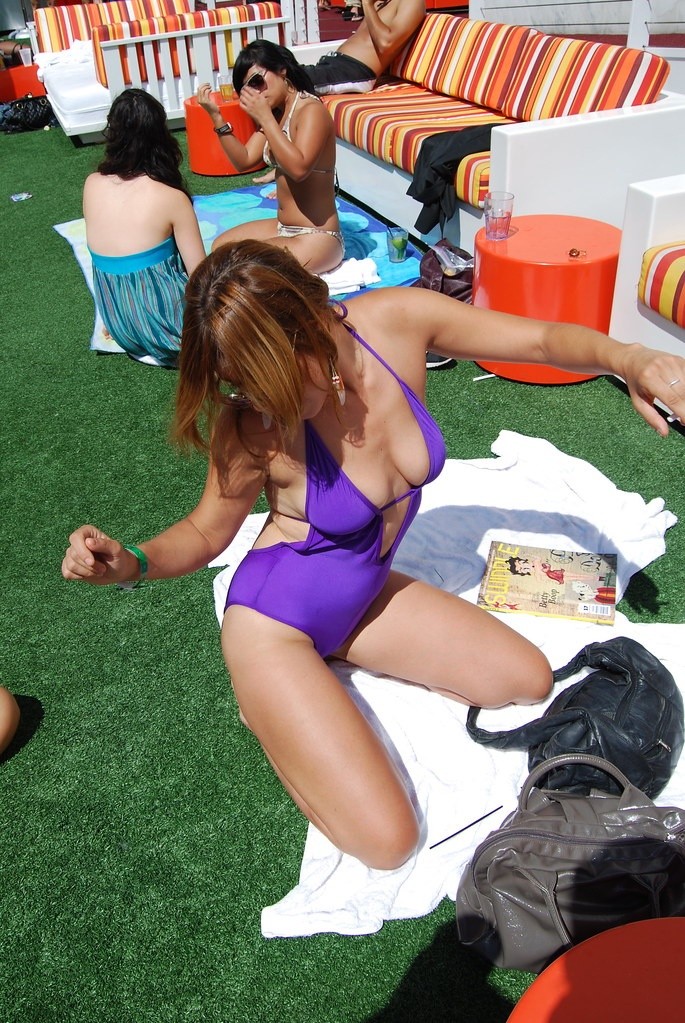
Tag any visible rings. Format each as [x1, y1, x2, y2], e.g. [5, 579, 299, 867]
[669, 379, 680, 387]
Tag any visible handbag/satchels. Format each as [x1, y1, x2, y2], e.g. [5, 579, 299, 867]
[0, 92, 55, 133]
[467, 636, 685, 795]
[409, 236, 475, 308]
[2, 43, 34, 68]
[453, 754, 685, 970]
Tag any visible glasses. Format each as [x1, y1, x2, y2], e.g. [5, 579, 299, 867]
[246, 68, 267, 89]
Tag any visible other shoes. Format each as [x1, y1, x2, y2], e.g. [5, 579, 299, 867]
[424, 352, 453, 368]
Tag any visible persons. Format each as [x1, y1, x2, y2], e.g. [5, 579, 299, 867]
[56, 239, 684, 869]
[82, 88, 210, 364]
[0, 40, 32, 58]
[251, 0, 427, 183]
[196, 37, 344, 272]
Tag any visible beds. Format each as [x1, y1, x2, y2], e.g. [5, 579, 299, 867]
[34, 0, 289, 148]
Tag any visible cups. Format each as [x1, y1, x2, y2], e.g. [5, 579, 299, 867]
[484, 191, 514, 241]
[386, 227, 409, 264]
[218, 75, 234, 103]
[19, 48, 32, 67]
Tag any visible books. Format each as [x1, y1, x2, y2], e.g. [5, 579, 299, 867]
[476, 540, 619, 625]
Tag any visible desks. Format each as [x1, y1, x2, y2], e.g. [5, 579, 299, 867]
[185, 90, 268, 175]
[473, 213, 624, 386]
[0, 62, 46, 103]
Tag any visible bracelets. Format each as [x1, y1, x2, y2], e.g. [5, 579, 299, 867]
[120, 546, 148, 589]
[214, 122, 234, 133]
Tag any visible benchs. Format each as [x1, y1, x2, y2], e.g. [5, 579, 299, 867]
[606, 174, 685, 431]
[286, 10, 684, 265]
